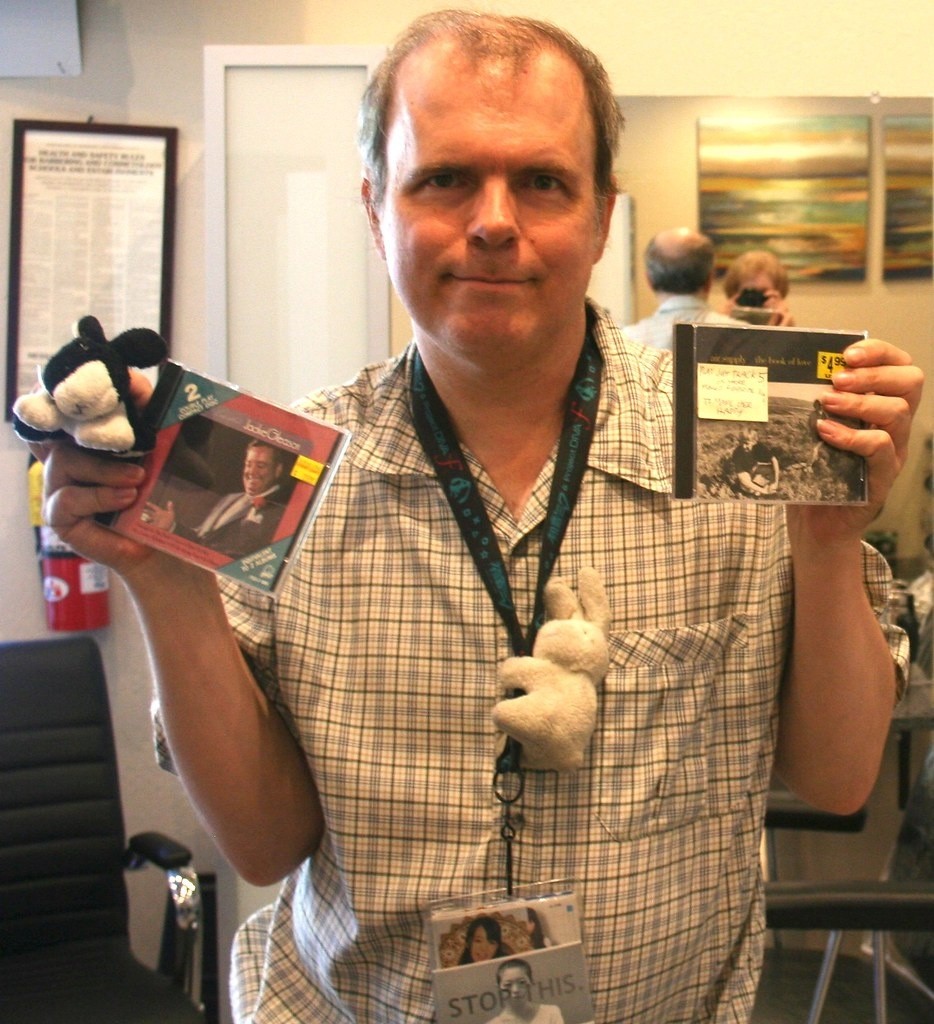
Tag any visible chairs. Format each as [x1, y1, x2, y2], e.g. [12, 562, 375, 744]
[890, 606, 934, 810]
[1, 635, 221, 1024]
[748, 741, 934, 1024]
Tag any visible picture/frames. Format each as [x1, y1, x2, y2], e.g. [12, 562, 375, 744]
[6, 119, 178, 426]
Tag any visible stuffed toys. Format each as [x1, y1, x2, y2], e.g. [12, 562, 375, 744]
[12, 315, 167, 464]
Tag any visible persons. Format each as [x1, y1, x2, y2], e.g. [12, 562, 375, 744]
[143, 438, 296, 559]
[892, 532, 934, 991]
[722, 400, 830, 494]
[618, 228, 799, 351]
[26, 8, 925, 1024]
[457, 908, 566, 1024]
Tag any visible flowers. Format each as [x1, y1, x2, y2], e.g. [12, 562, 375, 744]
[247, 496, 266, 524]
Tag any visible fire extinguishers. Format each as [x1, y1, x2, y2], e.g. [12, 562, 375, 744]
[27, 450, 114, 632]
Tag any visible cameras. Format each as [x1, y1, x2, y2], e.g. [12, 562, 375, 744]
[736, 289, 769, 307]
